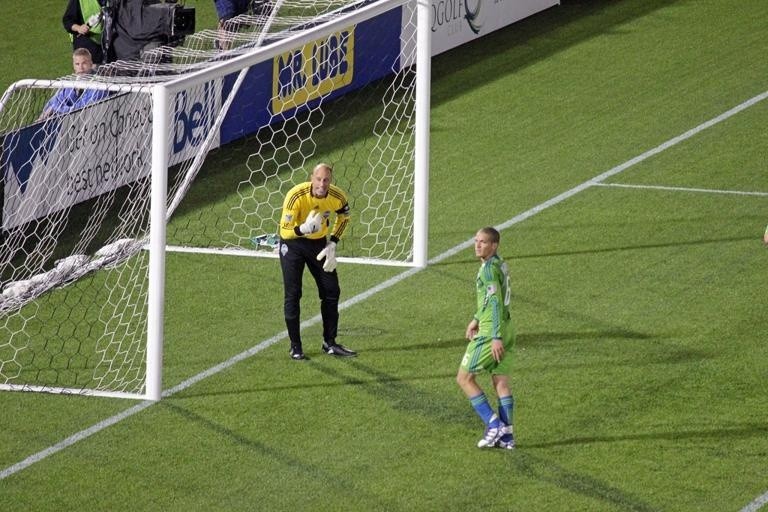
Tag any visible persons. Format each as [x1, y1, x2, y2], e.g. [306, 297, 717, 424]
[210, 1, 257, 55]
[456, 224, 517, 452]
[61, 1, 117, 70]
[277, 163, 359, 361]
[34, 48, 110, 121]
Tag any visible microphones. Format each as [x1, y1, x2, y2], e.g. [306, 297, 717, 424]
[76, 12, 103, 39]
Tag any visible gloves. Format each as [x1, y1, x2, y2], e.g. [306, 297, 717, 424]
[316, 240, 338, 273]
[299, 210, 323, 235]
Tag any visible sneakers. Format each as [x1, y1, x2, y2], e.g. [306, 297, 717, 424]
[477, 418, 516, 450]
[288, 347, 305, 360]
[321, 338, 358, 358]
